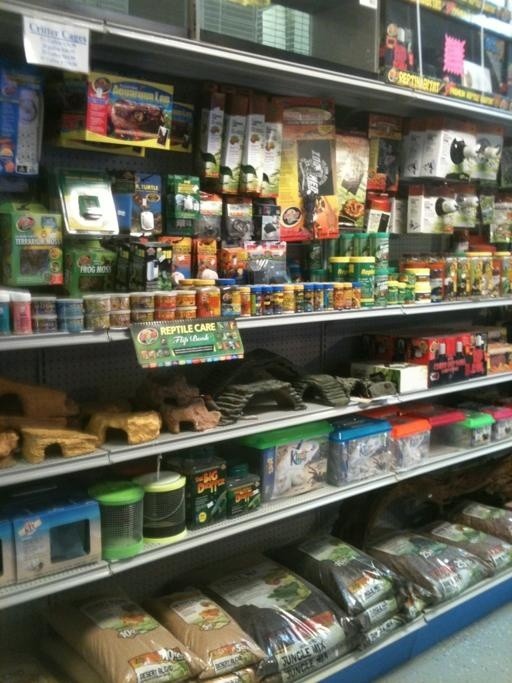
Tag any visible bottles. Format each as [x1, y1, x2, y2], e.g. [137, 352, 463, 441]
[87, 480, 145, 560]
[135, 470, 186, 544]
[0, 231, 512, 336]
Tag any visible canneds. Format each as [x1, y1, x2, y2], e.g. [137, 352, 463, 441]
[310, 232, 416, 308]
[154, 291, 177, 309]
[155, 308, 176, 320]
[131, 292, 155, 309]
[176, 280, 202, 290]
[32, 314, 58, 334]
[59, 316, 85, 333]
[131, 310, 154, 322]
[109, 310, 131, 328]
[0, 285, 33, 337]
[83, 294, 111, 314]
[231, 281, 361, 317]
[403, 251, 512, 303]
[56, 299, 84, 317]
[31, 293, 57, 315]
[176, 306, 198, 320]
[214, 279, 240, 320]
[84, 314, 111, 330]
[109, 293, 132, 310]
[175, 290, 197, 306]
[188, 280, 221, 318]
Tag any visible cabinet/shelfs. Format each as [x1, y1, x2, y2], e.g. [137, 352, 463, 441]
[0, 1, 110, 683]
[110, 0, 512, 683]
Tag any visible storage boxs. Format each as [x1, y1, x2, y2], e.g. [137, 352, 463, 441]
[352, 361, 428, 396]
[0, 519, 17, 587]
[483, 406, 512, 442]
[460, 411, 496, 447]
[404, 403, 466, 457]
[356, 406, 431, 473]
[60, 71, 175, 151]
[10, 485, 101, 585]
[364, 327, 488, 386]
[232, 420, 335, 502]
[326, 417, 393, 488]
